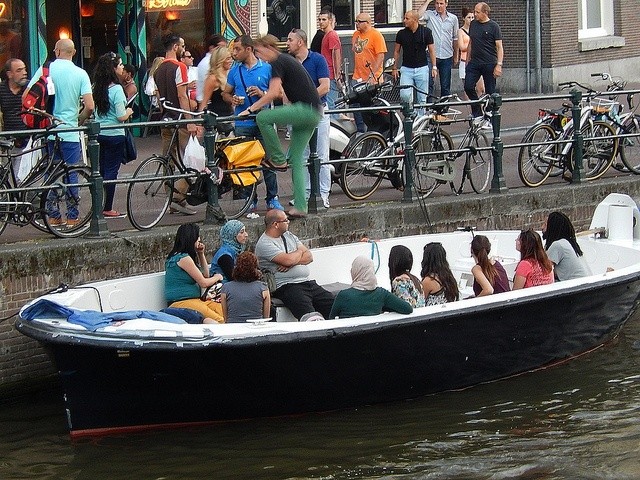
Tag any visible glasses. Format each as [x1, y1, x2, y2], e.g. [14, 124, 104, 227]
[276, 218, 288, 222]
[110, 52, 116, 61]
[291, 28, 305, 43]
[318, 18, 328, 21]
[355, 19, 369, 22]
[182, 56, 191, 58]
[177, 44, 184, 47]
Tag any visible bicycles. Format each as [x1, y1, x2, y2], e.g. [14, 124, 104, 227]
[518, 72, 639, 187]
[0, 108, 106, 238]
[399, 85, 492, 200]
[341, 85, 458, 201]
[127, 97, 255, 230]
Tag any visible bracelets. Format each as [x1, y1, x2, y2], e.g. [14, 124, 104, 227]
[432, 65, 438, 70]
[497, 62, 503, 67]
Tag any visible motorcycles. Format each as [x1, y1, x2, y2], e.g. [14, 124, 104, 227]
[285, 57, 403, 188]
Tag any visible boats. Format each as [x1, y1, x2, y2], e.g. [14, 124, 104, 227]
[15, 193, 640, 438]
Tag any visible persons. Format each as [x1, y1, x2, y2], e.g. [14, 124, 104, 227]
[286, 29, 331, 209]
[421, 242, 461, 306]
[91, 52, 135, 220]
[350, 12, 388, 137]
[464, 2, 505, 129]
[221, 251, 271, 323]
[177, 49, 198, 112]
[0, 58, 42, 223]
[237, 35, 323, 220]
[209, 219, 248, 284]
[255, 209, 336, 322]
[310, 12, 337, 54]
[458, 7, 487, 118]
[119, 64, 139, 103]
[415, 0, 459, 113]
[463, 234, 511, 299]
[388, 245, 426, 309]
[318, 10, 344, 123]
[153, 32, 199, 215]
[198, 46, 246, 201]
[21, 38, 95, 228]
[164, 222, 224, 324]
[391, 10, 438, 128]
[329, 256, 413, 318]
[145, 57, 164, 109]
[196, 34, 227, 111]
[512, 228, 555, 291]
[222, 34, 285, 216]
[541, 211, 591, 282]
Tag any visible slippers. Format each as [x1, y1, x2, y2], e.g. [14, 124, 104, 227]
[260, 159, 288, 172]
[286, 211, 308, 218]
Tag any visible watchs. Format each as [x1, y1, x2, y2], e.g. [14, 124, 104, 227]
[246, 106, 252, 114]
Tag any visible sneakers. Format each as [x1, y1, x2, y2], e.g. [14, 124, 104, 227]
[48, 217, 66, 227]
[240, 196, 256, 213]
[67, 219, 84, 229]
[473, 116, 483, 126]
[170, 198, 197, 216]
[267, 196, 285, 211]
[102, 209, 120, 215]
[480, 118, 494, 130]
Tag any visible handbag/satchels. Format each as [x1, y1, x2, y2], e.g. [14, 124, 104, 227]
[200, 281, 224, 302]
[21, 60, 55, 129]
[260, 268, 277, 294]
[122, 120, 137, 165]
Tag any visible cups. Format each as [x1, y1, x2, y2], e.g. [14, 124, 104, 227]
[235, 96, 245, 104]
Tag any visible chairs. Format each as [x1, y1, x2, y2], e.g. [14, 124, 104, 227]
[123, 91, 140, 137]
[141, 81, 167, 138]
